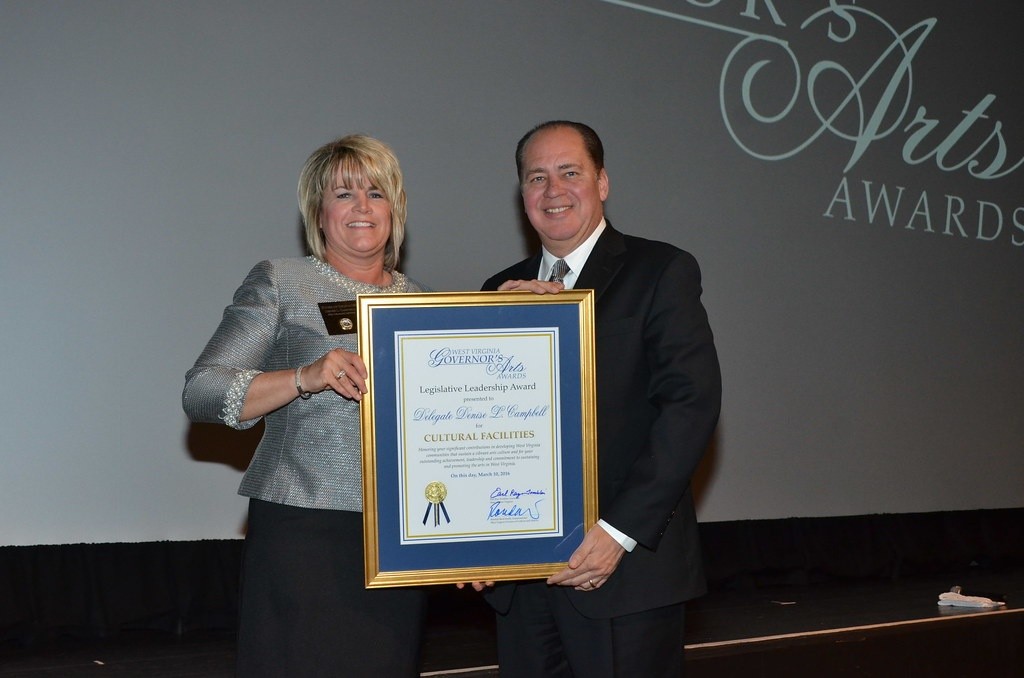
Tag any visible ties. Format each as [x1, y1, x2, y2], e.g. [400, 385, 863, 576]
[547, 259, 571, 286]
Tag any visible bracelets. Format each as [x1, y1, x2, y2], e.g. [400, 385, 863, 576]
[294, 364, 312, 400]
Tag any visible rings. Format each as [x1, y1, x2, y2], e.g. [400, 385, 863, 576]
[335, 370, 347, 381]
[589, 579, 597, 588]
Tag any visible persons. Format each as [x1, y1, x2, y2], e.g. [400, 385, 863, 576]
[479, 118, 723, 677]
[182, 134, 495, 678]
